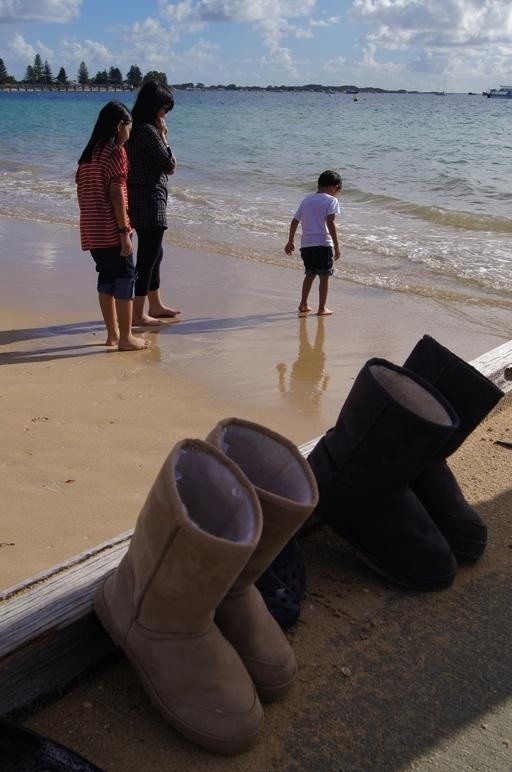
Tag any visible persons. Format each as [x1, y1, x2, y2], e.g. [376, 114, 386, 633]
[284, 170, 342, 316]
[74, 99, 151, 351]
[123, 80, 181, 325]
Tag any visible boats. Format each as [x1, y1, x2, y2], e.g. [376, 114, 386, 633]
[434, 91, 448, 96]
[486, 84, 512, 99]
[467, 92, 477, 95]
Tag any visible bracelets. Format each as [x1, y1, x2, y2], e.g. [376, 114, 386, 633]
[119, 226, 130, 234]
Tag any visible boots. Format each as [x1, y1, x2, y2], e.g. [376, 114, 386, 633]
[301, 354, 463, 593]
[398, 329, 507, 569]
[202, 400, 322, 695]
[96, 434, 265, 752]
